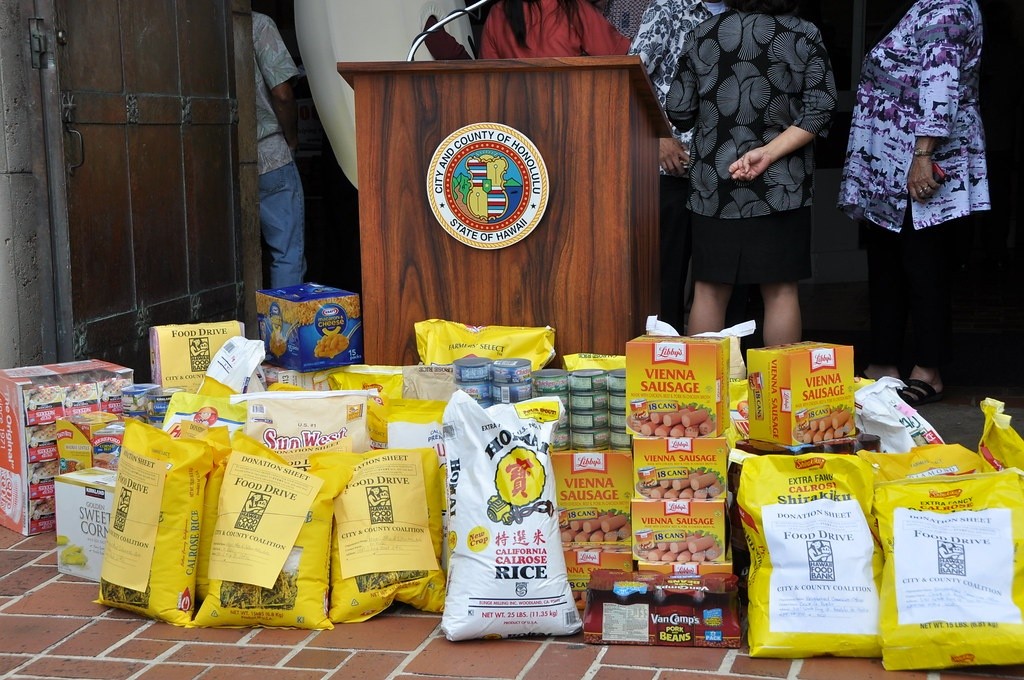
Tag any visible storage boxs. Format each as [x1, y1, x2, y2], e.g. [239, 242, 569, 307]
[548, 336, 858, 607]
[253, 280, 364, 392]
[0, 359, 135, 582]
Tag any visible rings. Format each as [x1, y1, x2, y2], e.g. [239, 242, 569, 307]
[922, 187, 929, 191]
[916, 190, 923, 195]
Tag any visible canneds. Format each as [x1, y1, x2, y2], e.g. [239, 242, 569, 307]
[453, 358, 632, 452]
[120, 385, 185, 430]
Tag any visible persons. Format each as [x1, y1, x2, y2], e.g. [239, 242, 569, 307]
[478, 0, 631, 60]
[666, 0, 837, 346]
[626, 0, 714, 335]
[252, 13, 307, 291]
[836, 0, 994, 405]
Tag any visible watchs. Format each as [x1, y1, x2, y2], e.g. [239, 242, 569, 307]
[913, 149, 933, 156]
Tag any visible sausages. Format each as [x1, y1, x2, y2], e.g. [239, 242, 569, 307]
[560, 405, 858, 563]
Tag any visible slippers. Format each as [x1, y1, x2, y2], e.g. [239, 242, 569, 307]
[897, 379, 942, 406]
[854, 370, 869, 379]
